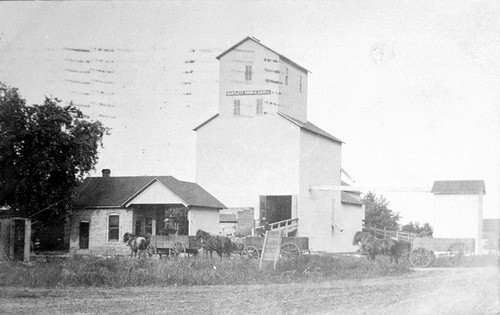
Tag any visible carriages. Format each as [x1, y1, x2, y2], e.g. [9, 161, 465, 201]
[123, 232, 203, 260]
[198, 229, 309, 263]
[353, 230, 474, 269]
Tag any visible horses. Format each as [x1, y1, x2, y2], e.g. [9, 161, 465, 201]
[195, 229, 232, 263]
[123, 232, 148, 259]
[352, 230, 401, 265]
